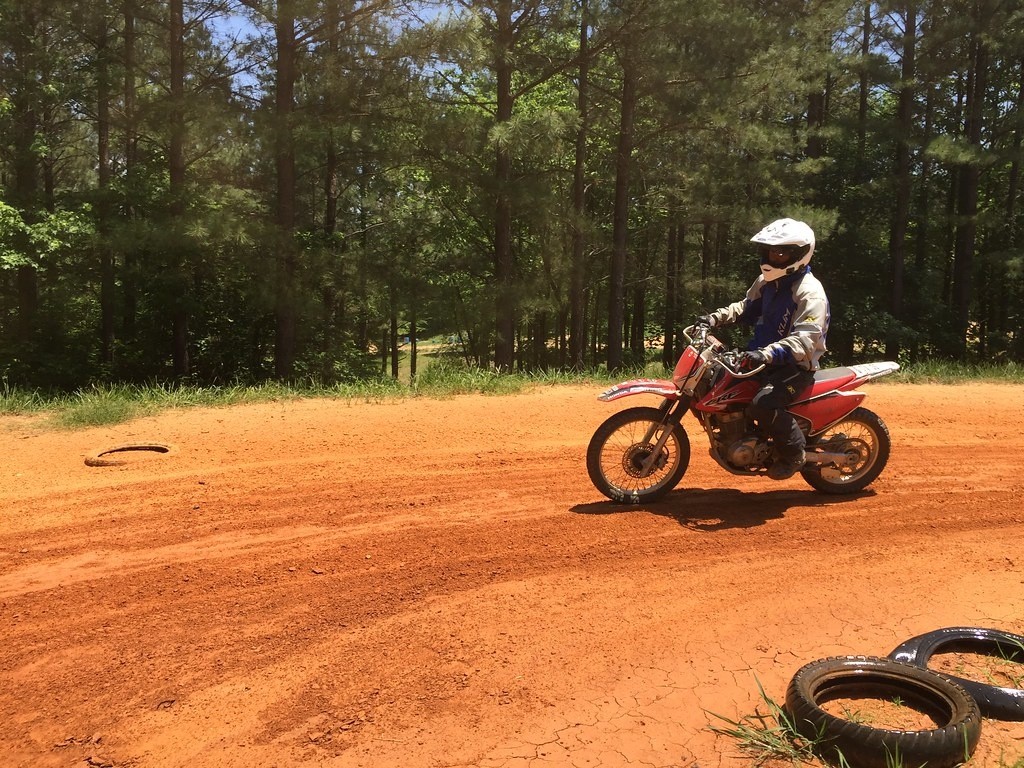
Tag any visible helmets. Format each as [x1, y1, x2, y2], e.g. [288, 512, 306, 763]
[749, 218, 815, 283]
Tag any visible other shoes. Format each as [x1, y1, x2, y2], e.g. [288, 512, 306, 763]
[765, 450, 806, 480]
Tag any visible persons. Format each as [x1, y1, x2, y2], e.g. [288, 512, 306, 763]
[695, 219, 829, 480]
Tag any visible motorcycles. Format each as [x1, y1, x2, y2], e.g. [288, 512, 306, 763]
[585, 316, 902, 507]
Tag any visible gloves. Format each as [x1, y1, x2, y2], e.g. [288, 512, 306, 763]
[691, 314, 712, 337]
[731, 349, 768, 372]
